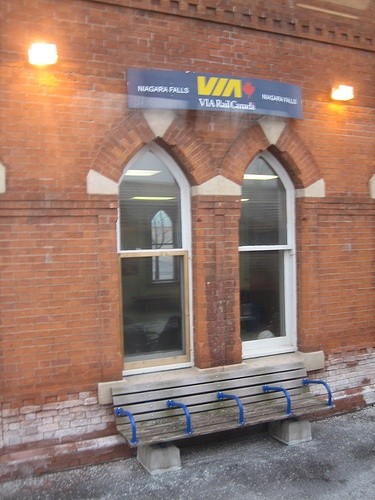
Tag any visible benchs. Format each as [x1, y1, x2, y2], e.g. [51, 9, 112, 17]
[110, 362, 336, 475]
